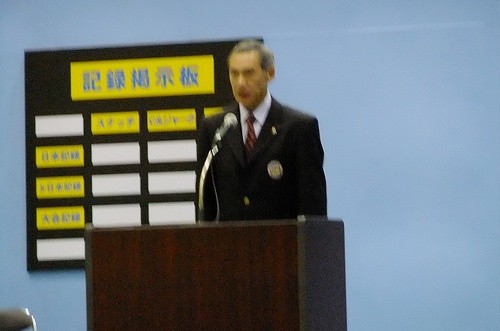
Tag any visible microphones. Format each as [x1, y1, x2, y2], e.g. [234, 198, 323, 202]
[213, 112, 238, 147]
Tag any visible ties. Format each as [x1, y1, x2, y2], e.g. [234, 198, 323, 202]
[244, 112, 256, 163]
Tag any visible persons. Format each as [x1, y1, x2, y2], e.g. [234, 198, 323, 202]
[194, 39, 327, 224]
